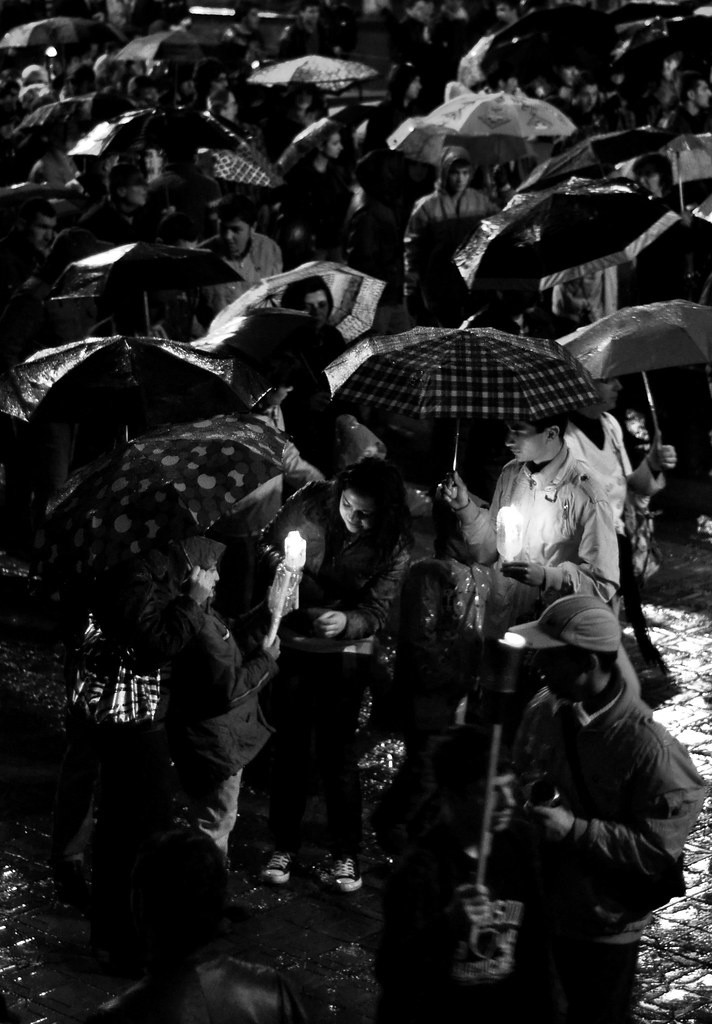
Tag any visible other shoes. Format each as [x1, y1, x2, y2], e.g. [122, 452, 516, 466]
[223, 905, 252, 925]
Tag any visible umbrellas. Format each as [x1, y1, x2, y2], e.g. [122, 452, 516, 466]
[617, 2, 709, 57]
[247, 55, 381, 92]
[420, 93, 579, 140]
[455, 3, 614, 93]
[5, 16, 123, 82]
[553, 298, 709, 451]
[320, 324, 607, 500]
[206, 259, 390, 385]
[35, 422, 291, 579]
[512, 129, 682, 192]
[202, 109, 287, 187]
[63, 104, 242, 159]
[40, 241, 249, 335]
[112, 27, 250, 67]
[4, 335, 276, 425]
[451, 174, 686, 293]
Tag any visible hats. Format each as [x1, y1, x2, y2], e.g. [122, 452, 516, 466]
[504, 592, 623, 654]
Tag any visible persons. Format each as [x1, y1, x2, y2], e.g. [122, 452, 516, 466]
[258, 453, 412, 894]
[566, 377, 683, 711]
[496, 593, 689, 1024]
[2, 1, 711, 399]
[434, 403, 622, 628]
[36, 538, 275, 981]
[83, 828, 308, 1024]
[374, 725, 571, 1022]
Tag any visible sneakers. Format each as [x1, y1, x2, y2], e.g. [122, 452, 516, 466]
[263, 851, 292, 884]
[334, 856, 363, 891]
